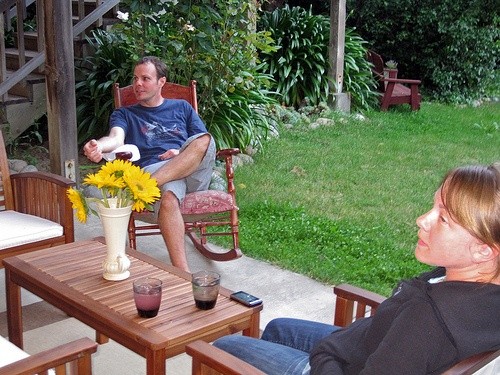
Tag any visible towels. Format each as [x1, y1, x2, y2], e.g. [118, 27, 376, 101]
[101, 143, 141, 163]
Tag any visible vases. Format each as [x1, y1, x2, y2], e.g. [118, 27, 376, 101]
[98, 198, 133, 281]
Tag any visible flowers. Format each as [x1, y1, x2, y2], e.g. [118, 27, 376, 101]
[66, 157, 161, 223]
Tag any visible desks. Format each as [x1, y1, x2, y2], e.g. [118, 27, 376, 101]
[0, 234, 265, 375]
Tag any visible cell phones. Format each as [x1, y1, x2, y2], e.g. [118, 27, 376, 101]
[230, 291, 262, 307]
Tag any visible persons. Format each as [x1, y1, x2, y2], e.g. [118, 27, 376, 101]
[83, 56, 216, 275]
[213, 163, 500, 374]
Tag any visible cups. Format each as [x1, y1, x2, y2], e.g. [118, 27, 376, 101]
[191, 270, 221, 310]
[132, 278, 162, 318]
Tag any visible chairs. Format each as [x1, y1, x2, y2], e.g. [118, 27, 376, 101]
[369, 52, 423, 113]
[185, 282, 499, 375]
[0, 130, 76, 318]
[115, 81, 243, 262]
[1, 334, 98, 375]
